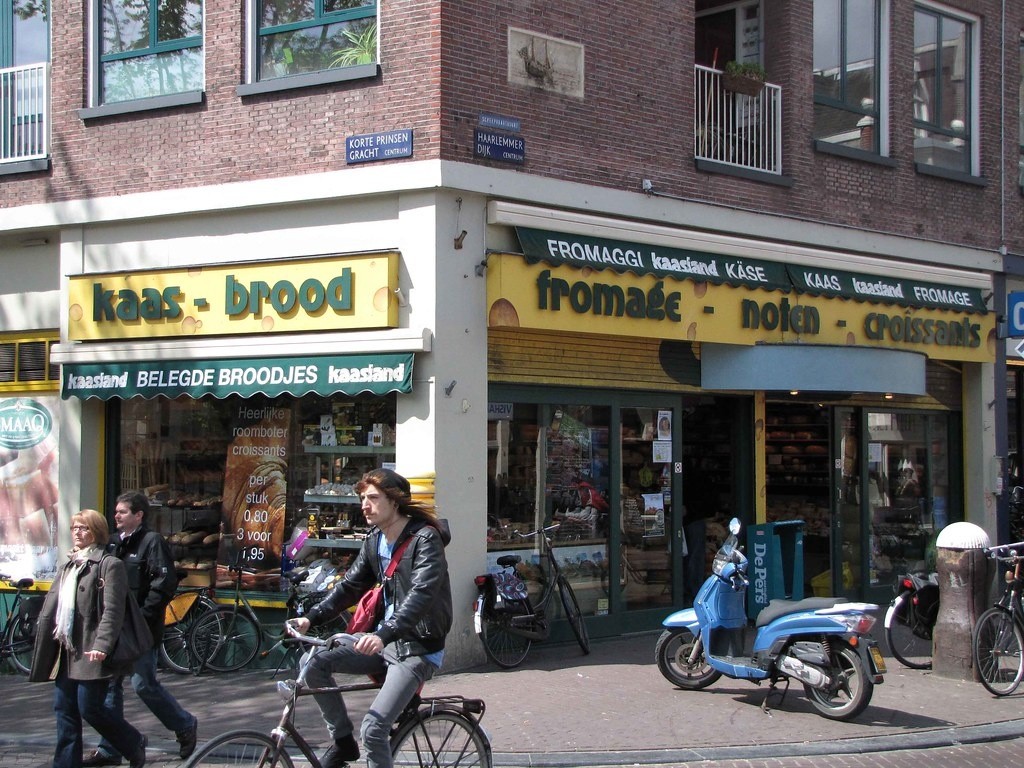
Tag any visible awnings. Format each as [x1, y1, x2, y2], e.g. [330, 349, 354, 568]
[47, 327, 432, 398]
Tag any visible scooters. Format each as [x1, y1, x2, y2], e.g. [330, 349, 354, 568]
[654, 518, 889, 722]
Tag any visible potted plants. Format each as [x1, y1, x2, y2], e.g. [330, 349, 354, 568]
[721, 60, 768, 97]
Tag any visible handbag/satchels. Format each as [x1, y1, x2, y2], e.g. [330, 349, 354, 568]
[344, 584, 384, 635]
[891, 574, 910, 627]
[19, 596, 45, 638]
[908, 574, 940, 640]
[481, 572, 528, 620]
[95, 554, 154, 668]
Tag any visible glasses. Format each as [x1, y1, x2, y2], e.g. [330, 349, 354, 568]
[70, 525, 89, 532]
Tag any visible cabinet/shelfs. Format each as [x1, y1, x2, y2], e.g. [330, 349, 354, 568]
[545, 426, 612, 541]
[140, 448, 229, 588]
[764, 423, 831, 487]
[302, 445, 395, 550]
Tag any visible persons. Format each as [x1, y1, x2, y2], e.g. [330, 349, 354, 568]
[26, 489, 198, 768]
[893, 464, 928, 498]
[681, 457, 707, 604]
[289, 471, 453, 767]
[570, 476, 609, 537]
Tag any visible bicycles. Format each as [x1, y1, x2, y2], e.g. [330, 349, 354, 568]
[971, 541, 1024, 698]
[186, 543, 353, 683]
[884, 574, 939, 670]
[470, 520, 590, 668]
[157, 568, 224, 677]
[183, 617, 493, 768]
[991, 485, 1024, 542]
[601, 539, 671, 598]
[0, 573, 47, 676]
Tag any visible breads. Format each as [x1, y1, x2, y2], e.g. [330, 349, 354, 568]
[142, 483, 223, 571]
[222, 406, 292, 560]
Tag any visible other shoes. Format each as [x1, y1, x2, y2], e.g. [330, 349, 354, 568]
[83, 750, 122, 767]
[312, 740, 360, 768]
[129, 734, 148, 768]
[176, 719, 197, 759]
